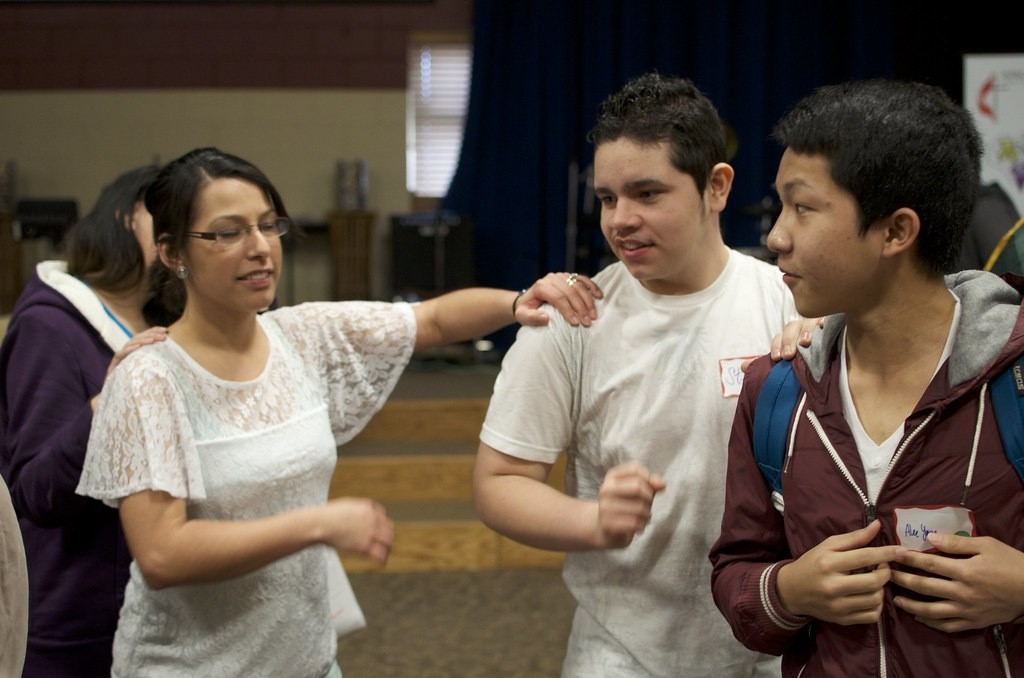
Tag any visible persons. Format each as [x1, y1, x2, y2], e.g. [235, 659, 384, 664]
[108, 142, 604, 677]
[708, 83, 1023, 677]
[0, 165, 169, 678]
[470, 69, 827, 678]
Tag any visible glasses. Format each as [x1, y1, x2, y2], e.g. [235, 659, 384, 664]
[177, 216, 291, 253]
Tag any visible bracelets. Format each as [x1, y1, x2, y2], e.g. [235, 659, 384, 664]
[512, 287, 531, 319]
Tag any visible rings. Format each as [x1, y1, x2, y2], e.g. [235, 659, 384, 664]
[566, 271, 579, 287]
